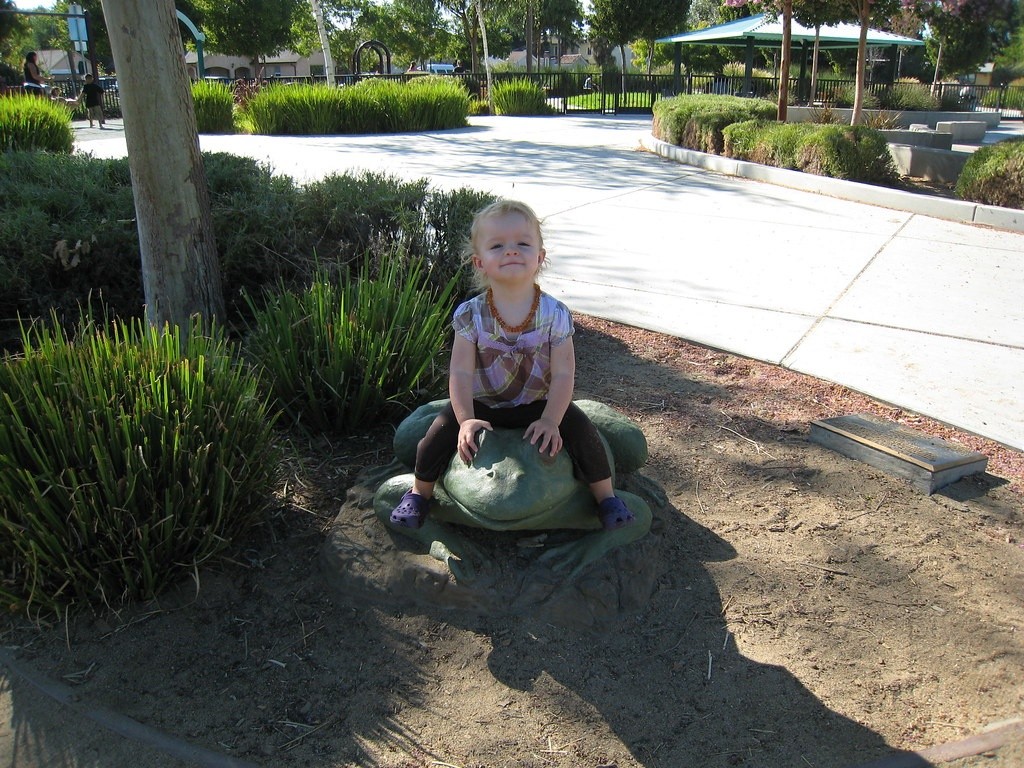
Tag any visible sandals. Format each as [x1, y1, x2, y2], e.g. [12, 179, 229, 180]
[597, 494, 635, 532]
[390, 486, 434, 529]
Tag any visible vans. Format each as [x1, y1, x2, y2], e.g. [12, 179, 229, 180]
[417, 64, 455, 74]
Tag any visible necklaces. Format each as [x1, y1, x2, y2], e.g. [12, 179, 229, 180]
[486, 284, 540, 332]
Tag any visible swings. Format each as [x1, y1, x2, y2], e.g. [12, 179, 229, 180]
[31, 20, 79, 109]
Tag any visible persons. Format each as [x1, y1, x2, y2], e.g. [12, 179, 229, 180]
[77, 74, 105, 128]
[407, 61, 419, 72]
[50, 87, 62, 101]
[24, 52, 51, 96]
[453, 60, 466, 73]
[584, 74, 596, 94]
[389, 200, 635, 531]
[954, 78, 976, 103]
